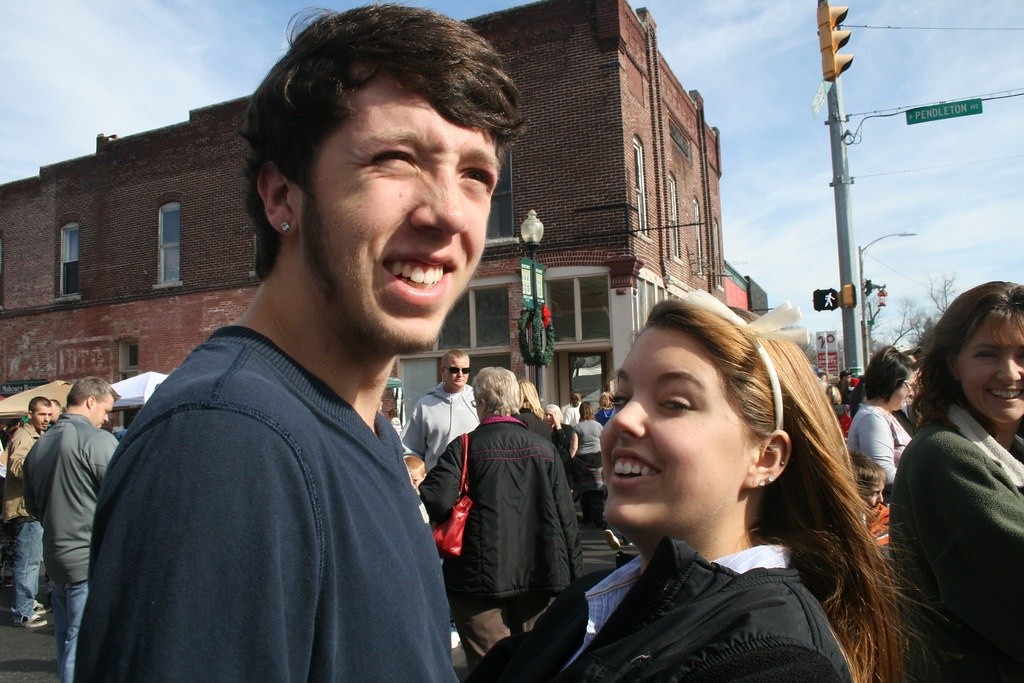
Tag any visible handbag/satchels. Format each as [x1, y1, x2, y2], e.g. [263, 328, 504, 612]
[431, 433, 473, 556]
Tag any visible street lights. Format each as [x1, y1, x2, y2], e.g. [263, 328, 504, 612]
[861, 230, 918, 370]
[520, 208, 546, 412]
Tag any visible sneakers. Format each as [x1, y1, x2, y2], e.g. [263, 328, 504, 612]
[14, 614, 48, 627]
[34, 603, 53, 614]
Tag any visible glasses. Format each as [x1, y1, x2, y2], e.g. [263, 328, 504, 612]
[903, 381, 918, 389]
[441, 367, 470, 374]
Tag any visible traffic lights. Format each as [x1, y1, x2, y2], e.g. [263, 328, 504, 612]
[813, 289, 838, 314]
[817, 0, 855, 83]
[837, 284, 858, 309]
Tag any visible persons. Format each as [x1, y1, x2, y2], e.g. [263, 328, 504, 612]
[71, 4, 533, 683]
[0, 280, 1024, 683]
[461, 296, 959, 683]
